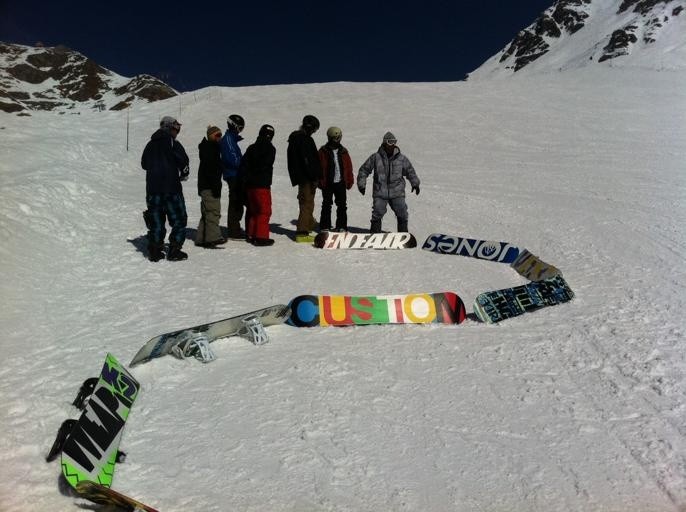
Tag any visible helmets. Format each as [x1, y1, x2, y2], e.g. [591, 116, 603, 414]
[227, 114, 245, 128]
[302, 115, 320, 128]
[326, 127, 342, 137]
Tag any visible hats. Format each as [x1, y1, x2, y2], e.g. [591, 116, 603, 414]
[259, 124, 275, 135]
[207, 126, 220, 136]
[383, 132, 397, 143]
[159, 116, 182, 130]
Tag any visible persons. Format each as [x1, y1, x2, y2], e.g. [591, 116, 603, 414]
[241, 123, 280, 248]
[139, 117, 189, 261]
[357, 131, 421, 233]
[287, 115, 322, 243]
[222, 114, 247, 242]
[198, 124, 227, 249]
[314, 127, 356, 235]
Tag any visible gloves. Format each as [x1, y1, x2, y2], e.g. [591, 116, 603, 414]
[411, 186, 420, 195]
[358, 187, 365, 195]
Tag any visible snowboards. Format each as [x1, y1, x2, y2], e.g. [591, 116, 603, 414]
[284, 291, 466, 325]
[64, 352, 140, 494]
[509, 247, 563, 283]
[75, 478, 158, 512]
[128, 303, 292, 369]
[421, 233, 519, 264]
[472, 275, 574, 324]
[314, 231, 417, 250]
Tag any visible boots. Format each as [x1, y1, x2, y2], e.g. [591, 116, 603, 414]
[148, 244, 188, 262]
[371, 224, 410, 232]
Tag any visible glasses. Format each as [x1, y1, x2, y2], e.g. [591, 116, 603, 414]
[384, 139, 397, 145]
[215, 133, 223, 137]
[329, 136, 341, 141]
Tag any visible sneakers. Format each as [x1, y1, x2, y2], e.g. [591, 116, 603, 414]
[320, 227, 351, 232]
[227, 229, 247, 238]
[248, 236, 275, 246]
[295, 232, 315, 243]
[195, 236, 228, 249]
